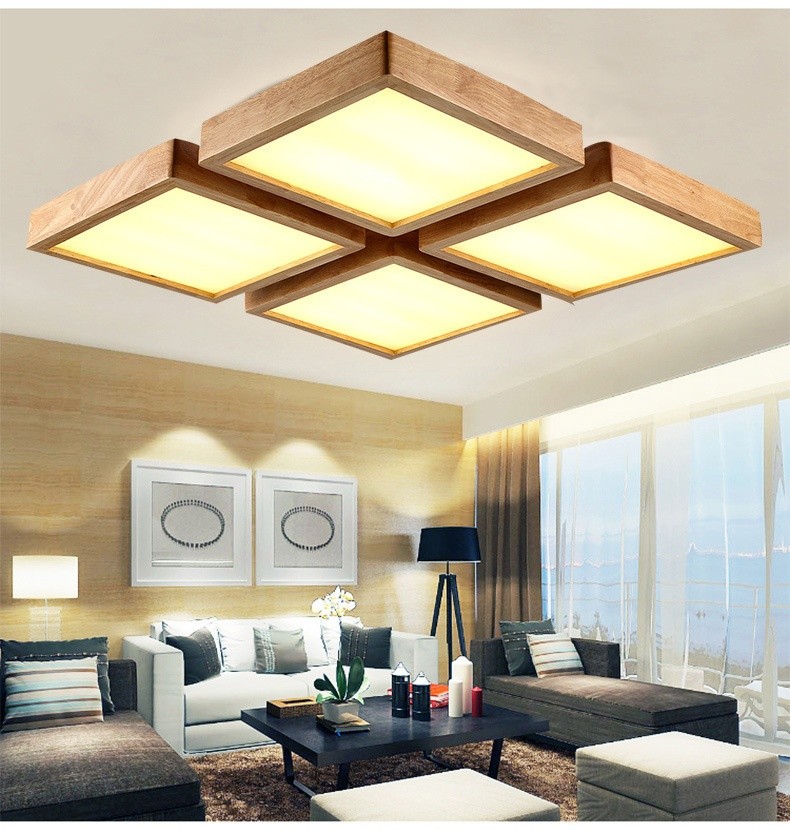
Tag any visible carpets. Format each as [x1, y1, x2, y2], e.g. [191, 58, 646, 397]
[182, 736, 790, 821]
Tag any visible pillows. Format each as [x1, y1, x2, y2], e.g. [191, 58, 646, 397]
[166, 628, 222, 690]
[252, 627, 310, 674]
[527, 634, 585, 679]
[498, 618, 555, 675]
[339, 623, 394, 671]
[0, 635, 115, 718]
[6, 656, 106, 731]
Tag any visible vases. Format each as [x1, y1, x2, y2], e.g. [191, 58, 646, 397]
[321, 701, 360, 723]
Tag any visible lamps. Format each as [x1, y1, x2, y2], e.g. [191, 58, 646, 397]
[13, 556, 79, 642]
[417, 526, 482, 680]
[26, 32, 761, 361]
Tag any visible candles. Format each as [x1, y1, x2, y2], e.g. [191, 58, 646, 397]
[392, 657, 483, 722]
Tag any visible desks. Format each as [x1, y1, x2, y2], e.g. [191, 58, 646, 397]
[241, 694, 550, 794]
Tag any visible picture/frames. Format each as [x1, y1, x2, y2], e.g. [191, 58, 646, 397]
[255, 470, 357, 585]
[131, 459, 252, 587]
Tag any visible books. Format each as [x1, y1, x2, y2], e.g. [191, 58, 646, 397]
[316, 715, 372, 734]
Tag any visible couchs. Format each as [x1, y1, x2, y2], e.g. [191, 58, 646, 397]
[121, 616, 440, 761]
[0, 660, 206, 821]
[470, 638, 739, 747]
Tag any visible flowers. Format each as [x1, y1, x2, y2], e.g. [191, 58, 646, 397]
[310, 584, 375, 706]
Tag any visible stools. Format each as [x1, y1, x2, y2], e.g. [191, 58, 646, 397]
[310, 766, 561, 822]
[576, 732, 778, 821]
